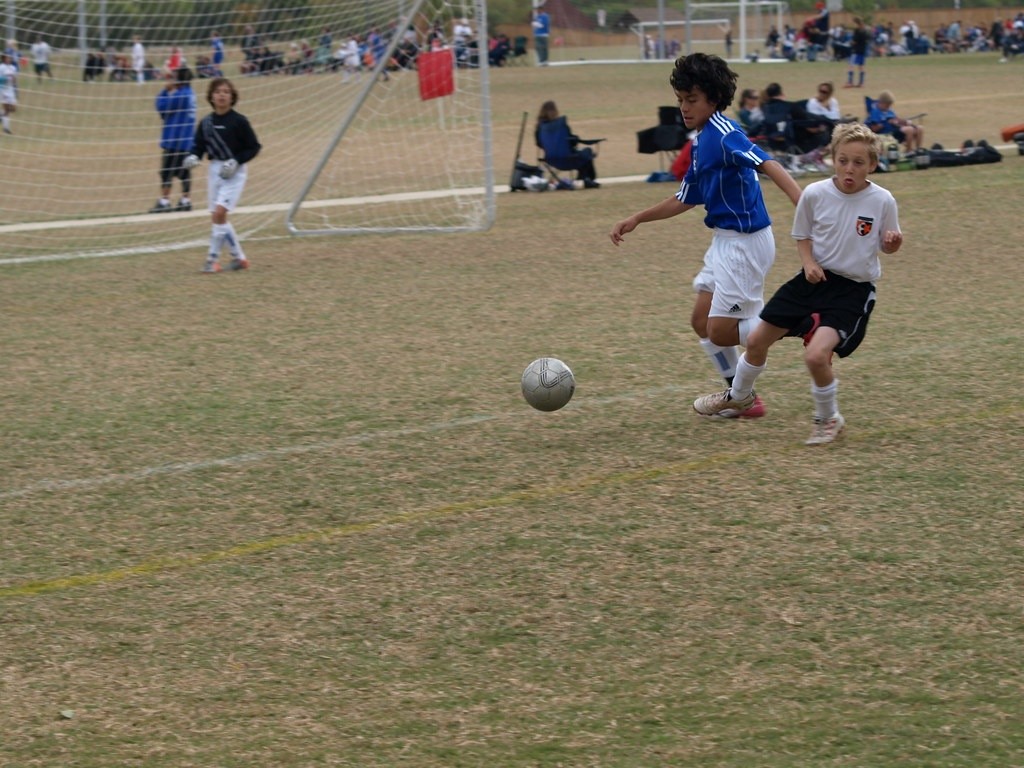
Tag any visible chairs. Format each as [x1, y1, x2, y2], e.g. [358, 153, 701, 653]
[866, 97, 926, 153]
[636, 105, 690, 173]
[741, 99, 860, 181]
[534, 124, 606, 192]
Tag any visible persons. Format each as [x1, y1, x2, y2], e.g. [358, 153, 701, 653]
[30, 34, 53, 85]
[182, 77, 263, 274]
[531, 7, 550, 67]
[83, 30, 226, 82]
[532, 101, 601, 187]
[725, 29, 733, 57]
[149, 67, 196, 213]
[692, 125, 904, 446]
[645, 34, 680, 59]
[239, 18, 510, 76]
[739, 82, 926, 152]
[0, 38, 22, 136]
[766, 0, 1024, 63]
[844, 15, 867, 89]
[747, 50, 760, 63]
[609, 52, 804, 425]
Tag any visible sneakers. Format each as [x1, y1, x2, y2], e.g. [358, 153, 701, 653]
[803, 312, 819, 348]
[806, 413, 845, 445]
[710, 396, 766, 418]
[693, 386, 755, 415]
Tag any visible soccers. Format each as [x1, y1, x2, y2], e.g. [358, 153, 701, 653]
[521, 358, 576, 412]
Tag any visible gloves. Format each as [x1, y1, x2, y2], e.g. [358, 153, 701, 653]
[219, 159, 239, 179]
[183, 154, 199, 170]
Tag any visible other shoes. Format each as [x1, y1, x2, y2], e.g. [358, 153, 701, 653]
[200, 262, 225, 273]
[150, 197, 172, 213]
[230, 257, 251, 270]
[2, 127, 12, 134]
[175, 198, 192, 212]
[584, 179, 601, 189]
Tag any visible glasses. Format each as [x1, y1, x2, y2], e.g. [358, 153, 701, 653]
[749, 96, 758, 100]
[819, 89, 828, 94]
